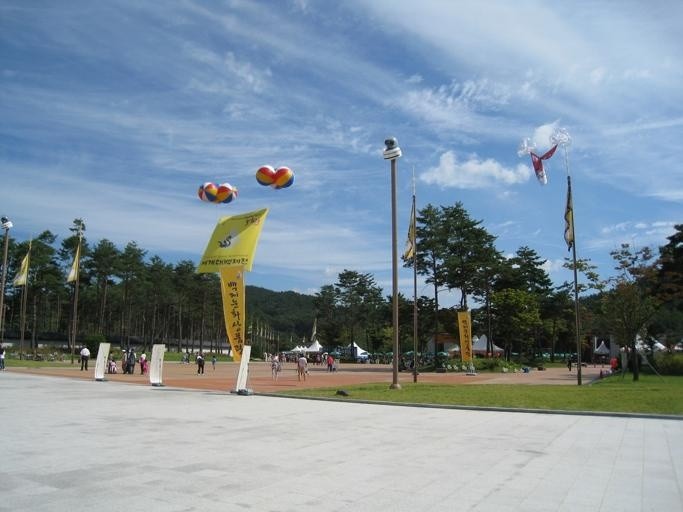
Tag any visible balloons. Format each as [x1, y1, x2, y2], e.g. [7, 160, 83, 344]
[197, 181, 238, 206]
[255, 165, 296, 190]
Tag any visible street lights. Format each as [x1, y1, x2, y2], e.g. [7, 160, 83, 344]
[383, 136, 401, 390]
[1, 217, 13, 325]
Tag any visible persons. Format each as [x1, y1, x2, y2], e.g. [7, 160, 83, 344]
[0, 348, 7, 371]
[183, 352, 205, 376]
[80, 345, 90, 371]
[566, 356, 572, 372]
[107, 348, 147, 376]
[262, 352, 337, 382]
[211, 356, 216, 370]
[610, 355, 618, 373]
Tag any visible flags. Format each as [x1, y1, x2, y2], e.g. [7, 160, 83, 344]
[564, 183, 575, 252]
[66, 243, 81, 284]
[402, 200, 416, 262]
[12, 251, 30, 288]
